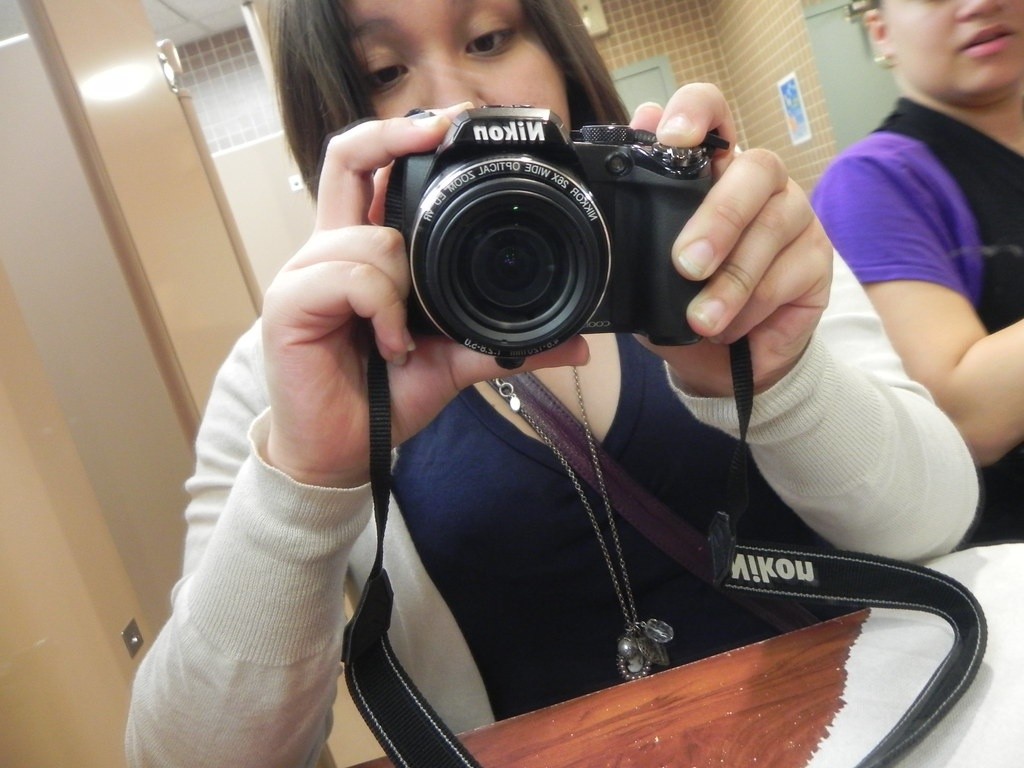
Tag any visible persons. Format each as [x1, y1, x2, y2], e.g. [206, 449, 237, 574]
[123, 0, 980, 768]
[810, 0, 1024, 549]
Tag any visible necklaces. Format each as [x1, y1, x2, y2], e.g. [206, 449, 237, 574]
[495, 366, 673, 680]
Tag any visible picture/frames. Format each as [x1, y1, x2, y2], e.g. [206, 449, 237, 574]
[776, 72, 812, 147]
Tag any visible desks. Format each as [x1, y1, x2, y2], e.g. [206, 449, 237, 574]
[355, 602, 867, 768]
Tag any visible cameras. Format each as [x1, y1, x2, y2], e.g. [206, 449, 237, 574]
[386, 107, 716, 370]
